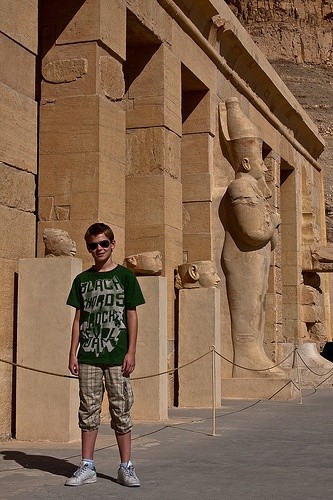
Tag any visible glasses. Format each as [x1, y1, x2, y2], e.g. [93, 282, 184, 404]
[87, 240, 112, 250]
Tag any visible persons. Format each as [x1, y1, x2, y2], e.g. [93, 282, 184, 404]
[178, 261, 221, 289]
[65, 222, 147, 486]
[222, 136, 287, 377]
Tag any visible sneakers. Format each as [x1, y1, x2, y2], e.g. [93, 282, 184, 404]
[116, 460, 141, 487]
[64, 461, 97, 486]
